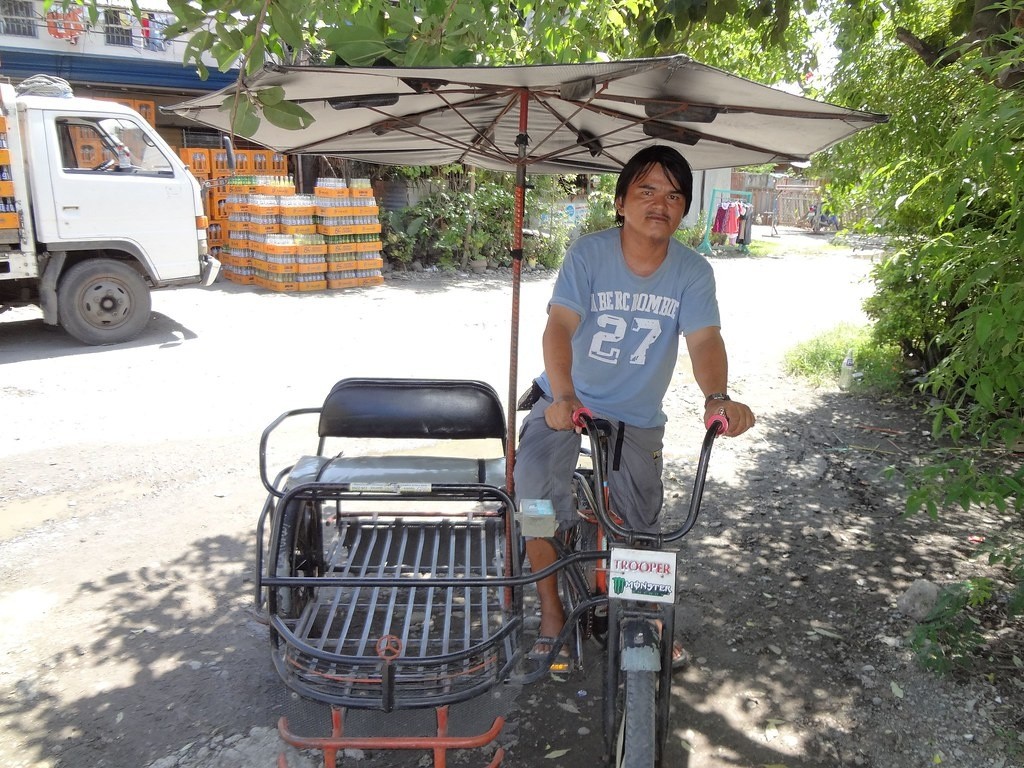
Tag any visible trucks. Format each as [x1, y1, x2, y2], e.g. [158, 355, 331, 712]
[0, 73, 235, 346]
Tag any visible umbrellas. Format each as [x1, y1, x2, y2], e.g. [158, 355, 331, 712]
[159, 54, 890, 608]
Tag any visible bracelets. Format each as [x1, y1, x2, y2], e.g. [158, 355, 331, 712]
[705, 393, 730, 408]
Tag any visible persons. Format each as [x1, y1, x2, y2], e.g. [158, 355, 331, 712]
[500, 146, 753, 670]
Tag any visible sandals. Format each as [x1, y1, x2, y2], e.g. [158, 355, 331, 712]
[658, 637, 689, 670]
[529, 623, 572, 661]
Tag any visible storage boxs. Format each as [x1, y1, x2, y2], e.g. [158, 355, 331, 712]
[180, 147, 384, 292]
[0, 116, 20, 244]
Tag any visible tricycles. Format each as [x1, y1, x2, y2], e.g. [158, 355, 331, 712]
[251, 376, 729, 768]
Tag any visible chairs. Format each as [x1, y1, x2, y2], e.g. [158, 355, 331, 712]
[285, 376, 509, 516]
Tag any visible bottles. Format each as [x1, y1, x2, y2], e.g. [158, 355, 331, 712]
[80, 127, 130, 168]
[839, 349, 854, 390]
[0, 133, 8, 149]
[0, 198, 15, 213]
[193, 153, 382, 282]
[0, 165, 12, 180]
[141, 105, 151, 110]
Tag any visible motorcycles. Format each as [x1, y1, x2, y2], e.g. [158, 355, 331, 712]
[807, 198, 839, 233]
